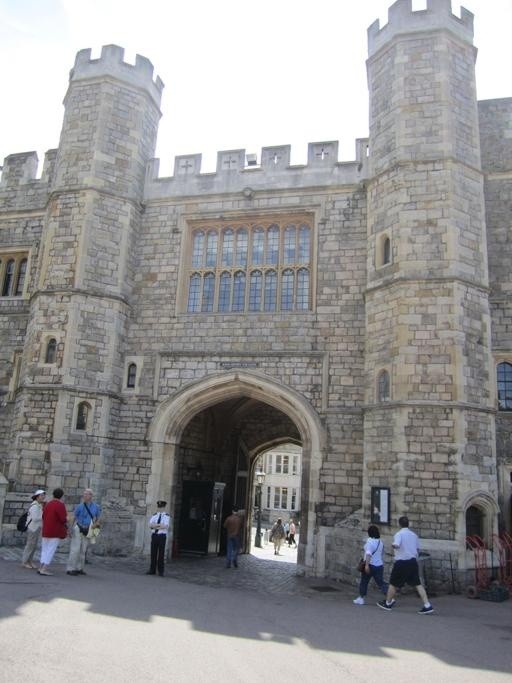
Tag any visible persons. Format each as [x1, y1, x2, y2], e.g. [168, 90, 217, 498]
[65, 488, 101, 576]
[270, 516, 297, 555]
[351, 524, 389, 605]
[377, 516, 434, 614]
[22, 490, 45, 568]
[147, 500, 170, 577]
[223, 506, 242, 569]
[37, 487, 68, 576]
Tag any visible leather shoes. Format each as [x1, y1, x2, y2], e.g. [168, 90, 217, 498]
[22, 562, 36, 568]
[73, 569, 86, 575]
[37, 568, 41, 572]
[40, 568, 54, 576]
[67, 569, 78, 576]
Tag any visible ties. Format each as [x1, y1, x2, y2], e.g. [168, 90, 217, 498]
[154, 513, 162, 534]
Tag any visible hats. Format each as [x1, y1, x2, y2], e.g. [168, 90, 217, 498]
[156, 500, 167, 508]
[31, 489, 46, 498]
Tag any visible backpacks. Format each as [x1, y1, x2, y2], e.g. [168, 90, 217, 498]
[16, 503, 36, 532]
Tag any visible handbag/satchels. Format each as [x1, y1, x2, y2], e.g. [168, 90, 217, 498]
[268, 525, 279, 542]
[356, 540, 381, 572]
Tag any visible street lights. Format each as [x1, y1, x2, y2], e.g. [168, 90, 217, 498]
[254, 464, 266, 546]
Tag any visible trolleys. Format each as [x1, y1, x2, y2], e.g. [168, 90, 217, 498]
[464, 532, 512, 605]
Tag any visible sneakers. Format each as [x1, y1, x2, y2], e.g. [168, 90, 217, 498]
[352, 596, 365, 605]
[376, 599, 395, 610]
[416, 603, 434, 614]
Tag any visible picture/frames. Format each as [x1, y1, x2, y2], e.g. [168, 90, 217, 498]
[370, 486, 391, 525]
